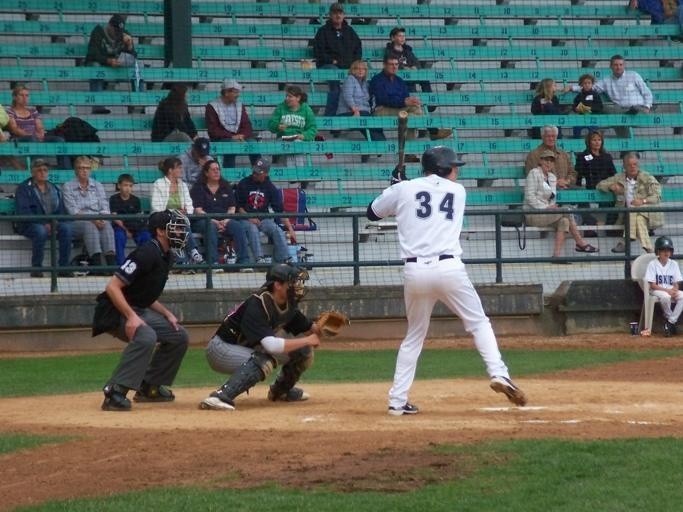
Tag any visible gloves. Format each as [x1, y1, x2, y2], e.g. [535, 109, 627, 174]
[390, 164, 408, 182]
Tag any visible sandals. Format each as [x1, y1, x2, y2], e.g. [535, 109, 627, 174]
[573, 244, 600, 252]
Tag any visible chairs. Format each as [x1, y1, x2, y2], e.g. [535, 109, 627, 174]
[627, 249, 682, 335]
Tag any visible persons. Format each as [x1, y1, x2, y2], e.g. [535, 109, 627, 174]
[177, 138, 216, 187]
[524, 150, 600, 265]
[368, 52, 453, 162]
[151, 83, 197, 142]
[528, 79, 564, 139]
[0, 84, 72, 170]
[14, 155, 296, 278]
[639, 237, 682, 337]
[269, 86, 317, 188]
[563, 54, 653, 158]
[596, 152, 662, 254]
[337, 61, 387, 163]
[526, 126, 587, 190]
[84, 14, 142, 114]
[91, 211, 191, 410]
[366, 146, 527, 416]
[206, 77, 261, 168]
[627, 0, 683, 68]
[197, 262, 350, 410]
[313, 3, 362, 118]
[383, 27, 437, 112]
[574, 132, 618, 238]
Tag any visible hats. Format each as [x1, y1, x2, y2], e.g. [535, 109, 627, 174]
[389, 26, 405, 36]
[30, 159, 51, 169]
[191, 137, 210, 157]
[329, 3, 345, 14]
[253, 156, 271, 176]
[222, 80, 244, 93]
[109, 15, 127, 32]
[539, 149, 556, 159]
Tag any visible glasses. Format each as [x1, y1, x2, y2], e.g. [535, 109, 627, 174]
[544, 156, 556, 162]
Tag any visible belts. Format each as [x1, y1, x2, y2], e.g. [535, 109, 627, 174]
[405, 255, 454, 263]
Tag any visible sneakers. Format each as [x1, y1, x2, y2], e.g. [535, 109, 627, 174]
[386, 403, 418, 417]
[613, 243, 628, 253]
[199, 396, 236, 410]
[584, 229, 598, 237]
[102, 385, 135, 412]
[663, 322, 675, 337]
[491, 375, 526, 405]
[605, 229, 618, 237]
[181, 249, 307, 273]
[268, 388, 309, 401]
[132, 385, 176, 402]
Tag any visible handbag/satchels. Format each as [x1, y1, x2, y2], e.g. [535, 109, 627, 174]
[501, 209, 523, 227]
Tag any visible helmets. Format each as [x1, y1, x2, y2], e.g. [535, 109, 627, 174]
[420, 145, 465, 177]
[260, 263, 309, 309]
[150, 209, 192, 248]
[653, 237, 674, 257]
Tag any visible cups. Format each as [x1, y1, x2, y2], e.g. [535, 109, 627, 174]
[301, 253, 314, 269]
[629, 321, 638, 336]
[264, 255, 271, 263]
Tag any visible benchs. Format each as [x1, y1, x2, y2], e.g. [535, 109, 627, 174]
[0, 1, 683, 254]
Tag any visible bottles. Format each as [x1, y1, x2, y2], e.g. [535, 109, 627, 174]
[226, 246, 235, 272]
[569, 150, 576, 166]
[581, 175, 586, 189]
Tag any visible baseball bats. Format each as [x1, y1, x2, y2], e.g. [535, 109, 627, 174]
[397, 110, 409, 172]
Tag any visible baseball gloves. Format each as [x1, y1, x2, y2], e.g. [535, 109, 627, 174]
[311, 311, 348, 338]
